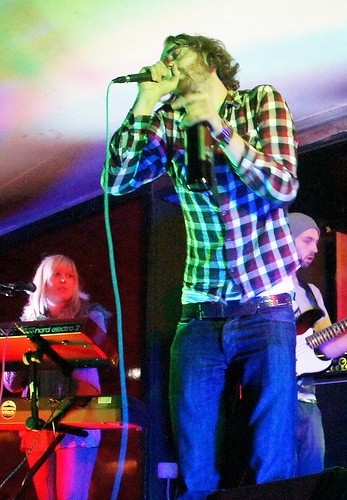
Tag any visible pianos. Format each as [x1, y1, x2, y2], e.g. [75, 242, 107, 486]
[0, 316, 152, 500]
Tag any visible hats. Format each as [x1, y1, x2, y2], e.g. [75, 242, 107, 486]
[287, 213, 320, 240]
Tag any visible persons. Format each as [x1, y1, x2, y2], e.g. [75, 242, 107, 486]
[99, 32, 302, 500]
[286, 212, 347, 478]
[3, 254, 111, 500]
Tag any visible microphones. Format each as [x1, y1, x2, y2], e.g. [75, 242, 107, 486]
[0, 283, 38, 297]
[112, 69, 173, 82]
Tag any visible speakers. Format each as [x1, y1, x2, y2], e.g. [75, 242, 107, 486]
[301, 373, 347, 470]
[0, 422, 145, 500]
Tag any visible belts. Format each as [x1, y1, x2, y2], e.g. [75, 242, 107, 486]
[181, 292, 292, 316]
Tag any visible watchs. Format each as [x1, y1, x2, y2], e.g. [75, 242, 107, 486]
[210, 119, 233, 148]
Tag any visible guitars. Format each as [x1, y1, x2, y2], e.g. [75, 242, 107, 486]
[295, 318, 347, 378]
